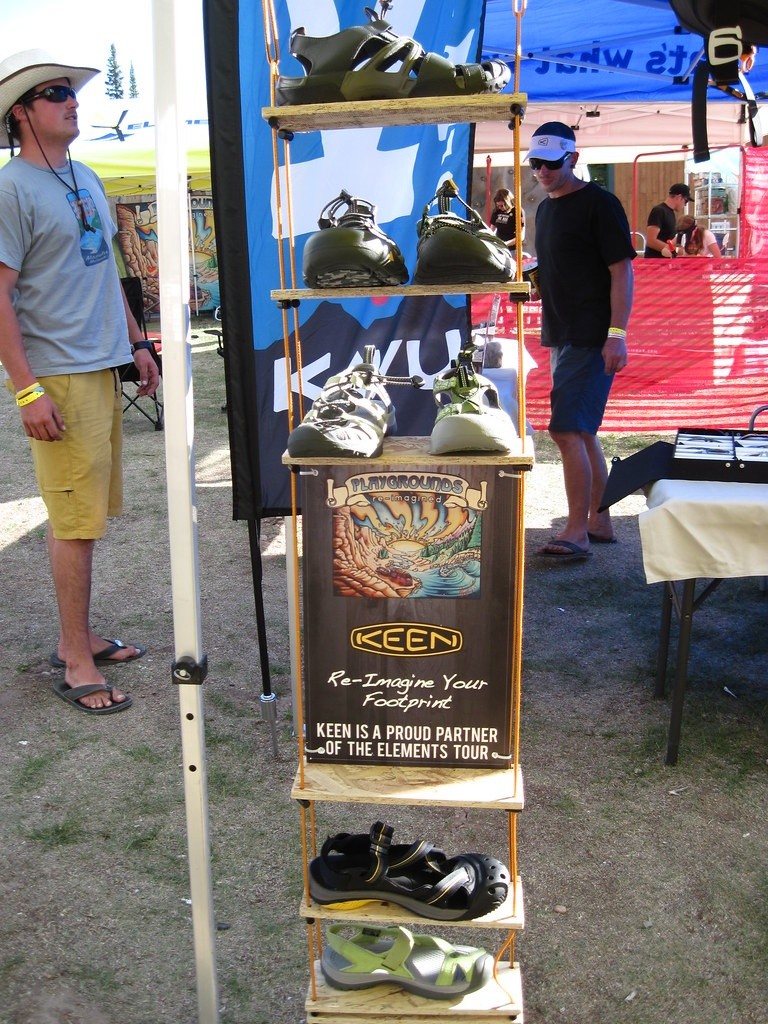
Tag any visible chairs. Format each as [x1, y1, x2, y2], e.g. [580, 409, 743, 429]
[117, 276, 163, 431]
[204, 307, 228, 411]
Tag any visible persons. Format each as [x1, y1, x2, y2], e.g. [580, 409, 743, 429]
[644, 183, 695, 258]
[490, 188, 526, 265]
[0, 56, 159, 717]
[671, 215, 722, 258]
[509, 120, 638, 559]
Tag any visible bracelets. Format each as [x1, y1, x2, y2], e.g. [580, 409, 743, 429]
[608, 327, 626, 341]
[15, 382, 45, 406]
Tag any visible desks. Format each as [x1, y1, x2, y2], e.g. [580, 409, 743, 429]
[638, 477, 768, 765]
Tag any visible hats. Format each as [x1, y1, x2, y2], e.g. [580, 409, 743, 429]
[669, 184, 696, 202]
[1, 49, 100, 149]
[522, 135, 577, 162]
[675, 219, 697, 245]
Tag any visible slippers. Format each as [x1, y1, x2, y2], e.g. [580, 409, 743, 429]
[533, 540, 594, 558]
[53, 678, 133, 715]
[51, 638, 145, 667]
[588, 533, 617, 543]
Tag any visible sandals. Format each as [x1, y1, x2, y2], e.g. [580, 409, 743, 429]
[272, 0, 511, 106]
[300, 192, 409, 286]
[287, 344, 396, 459]
[412, 180, 518, 285]
[306, 819, 509, 921]
[320, 922, 495, 1000]
[432, 340, 518, 454]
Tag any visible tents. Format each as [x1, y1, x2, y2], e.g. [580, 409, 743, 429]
[149, 0, 767, 1024]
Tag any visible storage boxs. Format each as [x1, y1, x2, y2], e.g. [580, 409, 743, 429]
[597, 428, 768, 514]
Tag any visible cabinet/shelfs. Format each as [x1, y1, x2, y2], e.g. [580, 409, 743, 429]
[694, 172, 740, 258]
[263, 0, 538, 1023]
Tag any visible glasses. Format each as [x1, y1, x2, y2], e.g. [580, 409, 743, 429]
[684, 197, 688, 203]
[22, 86, 76, 105]
[528, 152, 571, 170]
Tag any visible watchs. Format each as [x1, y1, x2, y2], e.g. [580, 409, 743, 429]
[131, 341, 154, 354]
